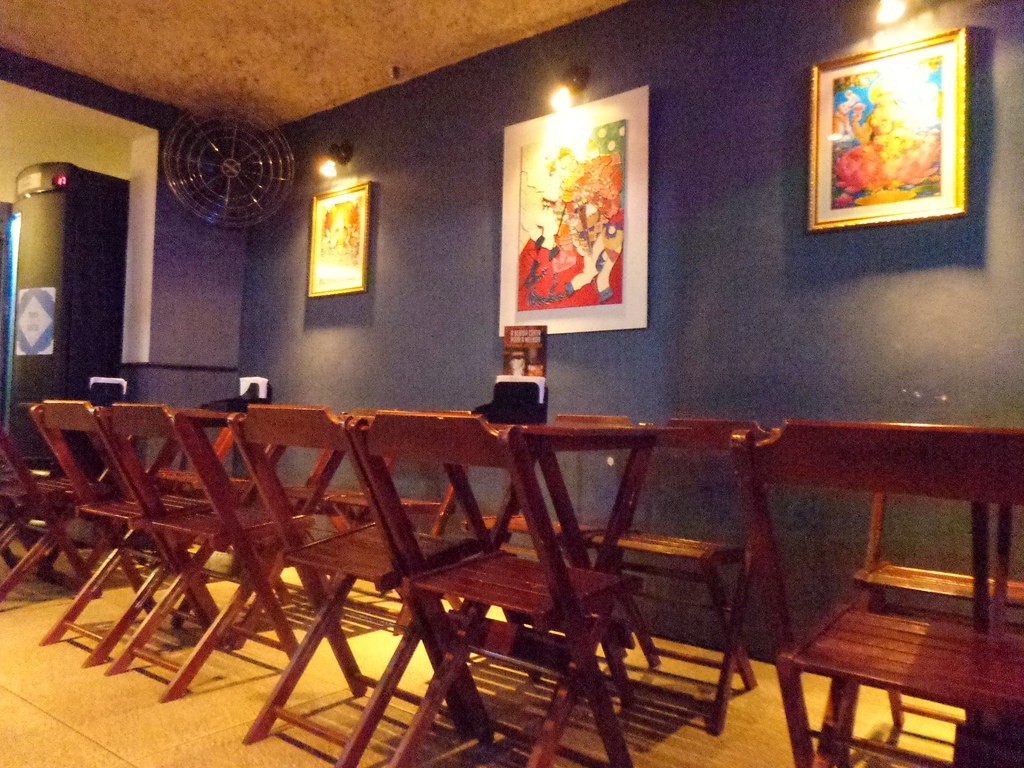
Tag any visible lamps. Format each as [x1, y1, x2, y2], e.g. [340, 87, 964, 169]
[549, 64, 591, 114]
[318, 141, 353, 177]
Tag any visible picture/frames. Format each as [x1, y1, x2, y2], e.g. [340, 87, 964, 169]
[308, 181, 371, 298]
[808, 25, 969, 231]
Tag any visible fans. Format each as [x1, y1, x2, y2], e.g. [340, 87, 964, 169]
[163, 102, 295, 229]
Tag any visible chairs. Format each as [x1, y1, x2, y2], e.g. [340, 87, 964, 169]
[1, 401, 1024, 768]
